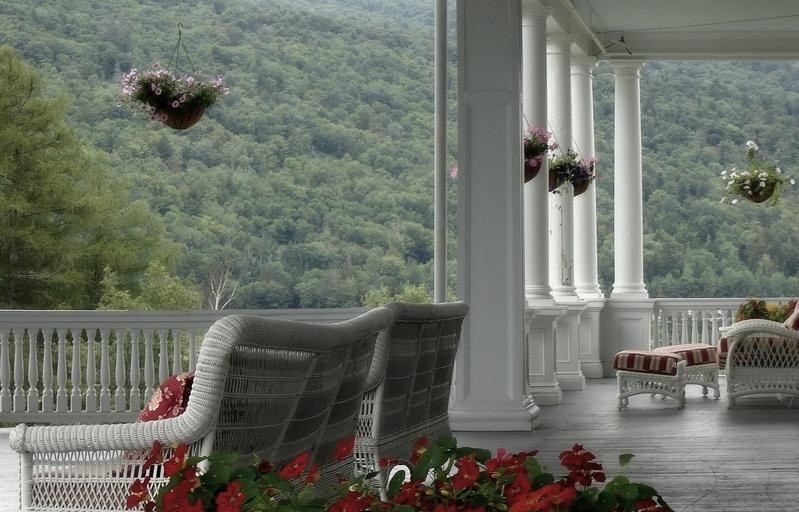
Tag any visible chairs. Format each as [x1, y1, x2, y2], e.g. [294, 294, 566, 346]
[0, 301, 394, 511]
[714, 298, 799, 411]
[351, 299, 470, 506]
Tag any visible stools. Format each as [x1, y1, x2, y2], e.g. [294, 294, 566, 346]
[654, 342, 720, 400]
[612, 350, 688, 411]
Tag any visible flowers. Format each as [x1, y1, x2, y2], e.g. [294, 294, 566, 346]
[448, 122, 555, 185]
[577, 152, 603, 188]
[716, 136, 797, 214]
[113, 60, 230, 133]
[543, 148, 579, 197]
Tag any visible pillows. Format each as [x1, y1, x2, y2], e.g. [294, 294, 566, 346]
[120, 372, 193, 480]
